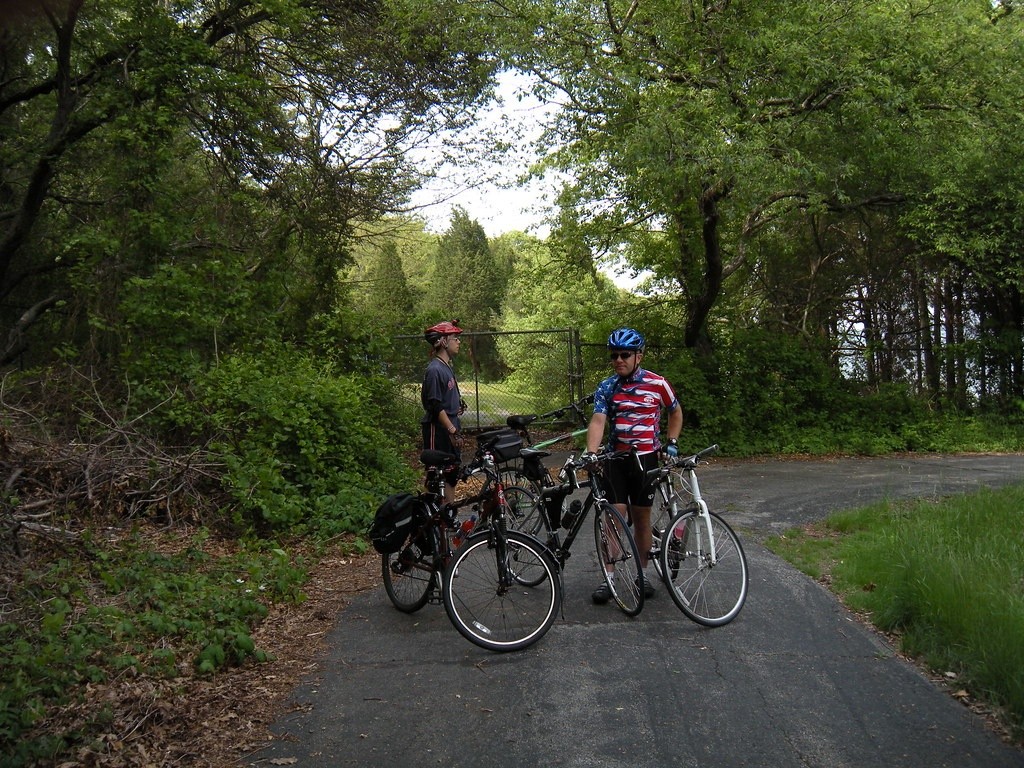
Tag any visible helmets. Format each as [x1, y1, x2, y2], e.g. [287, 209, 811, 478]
[608, 328, 645, 352]
[424, 321, 463, 346]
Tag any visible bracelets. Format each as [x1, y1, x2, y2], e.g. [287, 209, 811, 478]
[447, 425, 454, 431]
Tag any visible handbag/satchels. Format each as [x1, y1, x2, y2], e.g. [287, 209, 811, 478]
[370, 490, 435, 557]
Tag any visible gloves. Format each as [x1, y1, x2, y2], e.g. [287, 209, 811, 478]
[659, 437, 678, 462]
[582, 451, 596, 471]
[449, 430, 465, 449]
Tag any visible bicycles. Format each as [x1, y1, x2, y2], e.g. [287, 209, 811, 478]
[493, 446, 647, 617]
[638, 443, 750, 628]
[479, 389, 634, 549]
[381, 435, 561, 653]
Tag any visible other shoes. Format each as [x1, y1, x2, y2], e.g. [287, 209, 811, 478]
[635, 574, 655, 598]
[450, 517, 460, 532]
[592, 579, 616, 603]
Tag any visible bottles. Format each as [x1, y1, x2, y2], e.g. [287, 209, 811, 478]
[674, 510, 686, 540]
[558, 453, 576, 481]
[451, 515, 477, 548]
[561, 499, 582, 530]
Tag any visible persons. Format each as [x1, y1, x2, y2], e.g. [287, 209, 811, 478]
[580, 329, 682, 606]
[421, 321, 464, 532]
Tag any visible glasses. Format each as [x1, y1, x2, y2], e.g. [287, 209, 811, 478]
[610, 352, 638, 360]
[449, 338, 460, 341]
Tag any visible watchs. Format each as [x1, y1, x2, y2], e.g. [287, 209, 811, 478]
[666, 437, 679, 445]
